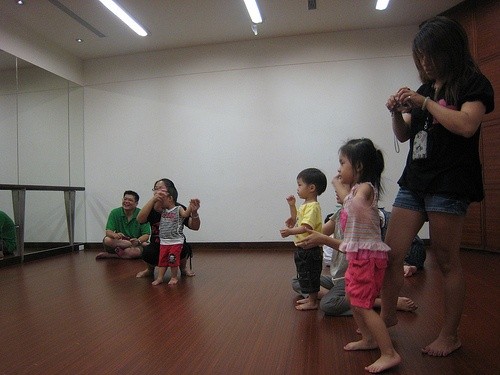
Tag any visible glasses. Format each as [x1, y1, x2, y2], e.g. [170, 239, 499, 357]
[123, 197, 135, 202]
[152, 188, 168, 192]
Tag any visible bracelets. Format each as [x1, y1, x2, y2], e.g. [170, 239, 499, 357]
[191, 214, 199, 219]
[422, 96, 430, 111]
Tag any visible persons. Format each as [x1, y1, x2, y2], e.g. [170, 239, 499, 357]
[356, 16, 495, 356]
[154, 188, 200, 285]
[96, 190, 151, 259]
[280, 167, 328, 310]
[136, 178, 201, 278]
[0, 210, 16, 257]
[331, 137, 402, 373]
[291, 174, 426, 316]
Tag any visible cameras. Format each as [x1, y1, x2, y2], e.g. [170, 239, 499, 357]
[393, 100, 412, 111]
[122, 236, 130, 241]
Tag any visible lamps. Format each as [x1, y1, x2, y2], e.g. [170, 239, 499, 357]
[100, 0, 151, 37]
[243, 0, 265, 24]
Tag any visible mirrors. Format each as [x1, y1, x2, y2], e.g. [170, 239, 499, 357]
[0, 49, 87, 267]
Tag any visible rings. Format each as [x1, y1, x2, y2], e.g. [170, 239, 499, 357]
[408, 96, 410, 100]
